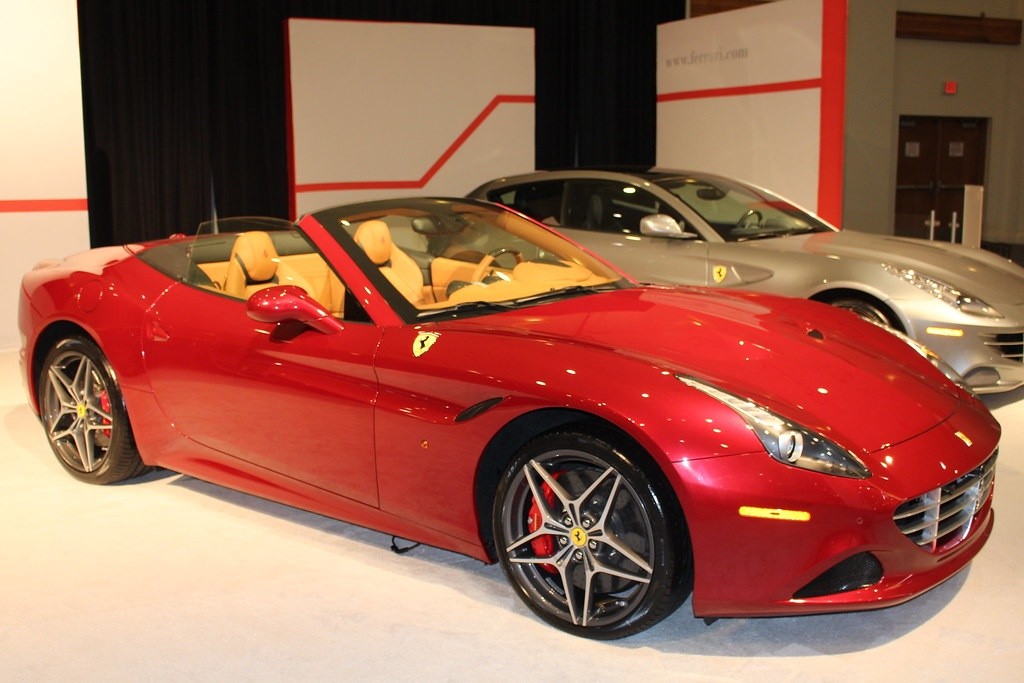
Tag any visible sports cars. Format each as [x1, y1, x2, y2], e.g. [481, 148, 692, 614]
[463, 170, 1023, 400]
[15, 189, 1001, 644]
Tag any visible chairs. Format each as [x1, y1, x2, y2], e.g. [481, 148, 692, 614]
[329, 218, 424, 318]
[585, 195, 625, 232]
[222, 231, 317, 311]
